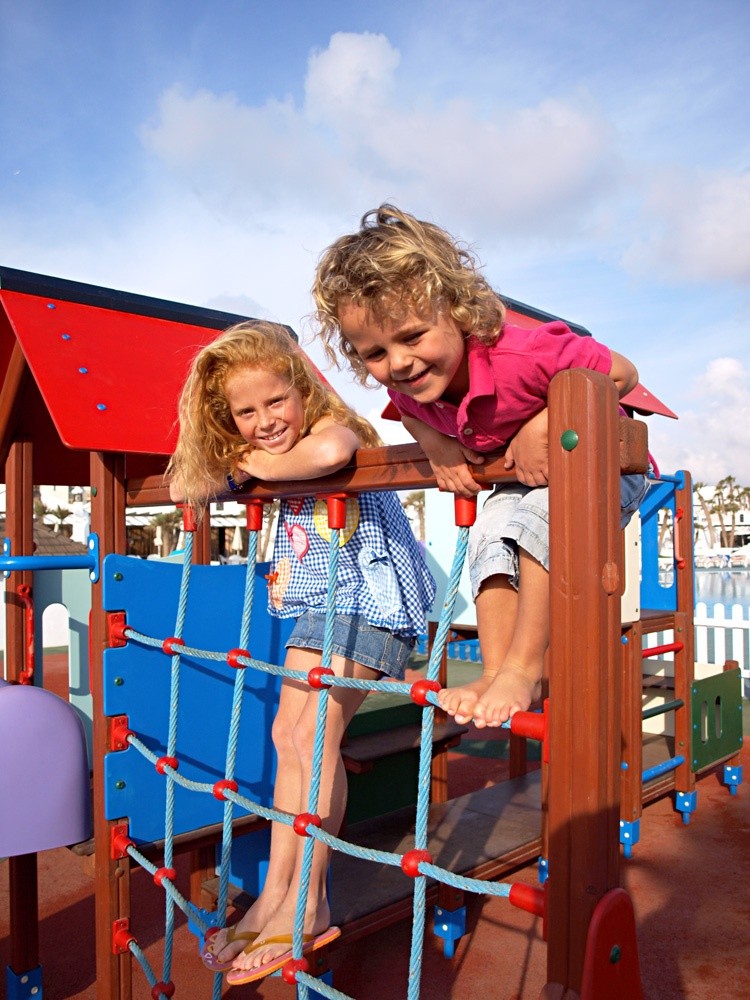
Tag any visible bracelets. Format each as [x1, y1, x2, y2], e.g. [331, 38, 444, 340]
[226, 470, 243, 490]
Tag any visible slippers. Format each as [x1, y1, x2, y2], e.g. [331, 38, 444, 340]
[226, 926, 341, 985]
[201, 921, 260, 972]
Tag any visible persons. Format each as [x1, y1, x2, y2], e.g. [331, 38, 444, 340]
[160, 320, 438, 986]
[310, 201, 652, 728]
